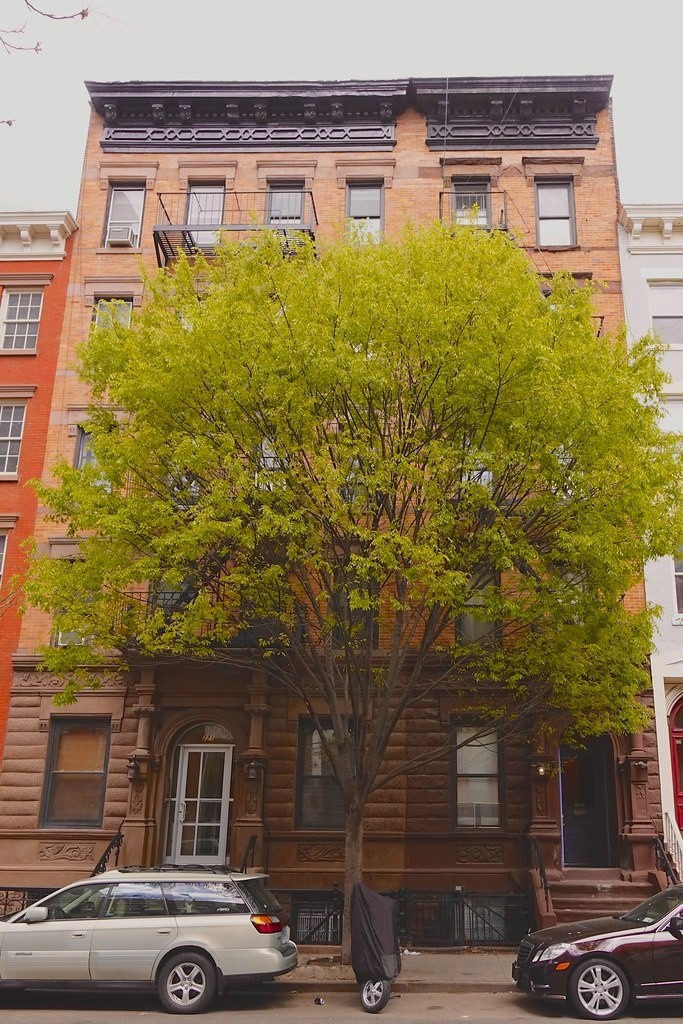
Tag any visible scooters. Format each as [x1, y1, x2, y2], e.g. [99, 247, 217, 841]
[351, 882, 402, 1014]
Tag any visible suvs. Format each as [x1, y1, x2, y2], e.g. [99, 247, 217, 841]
[0, 868, 301, 1017]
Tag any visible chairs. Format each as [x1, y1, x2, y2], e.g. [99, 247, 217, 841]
[128, 893, 146, 916]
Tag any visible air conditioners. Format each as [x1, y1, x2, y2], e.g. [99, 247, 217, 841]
[107, 226, 135, 248]
[57, 628, 87, 647]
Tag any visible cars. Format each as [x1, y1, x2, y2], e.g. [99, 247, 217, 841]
[510, 882, 683, 1021]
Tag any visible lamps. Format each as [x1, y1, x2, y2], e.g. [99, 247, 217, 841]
[126, 755, 140, 781]
[247, 753, 258, 779]
[531, 754, 555, 776]
